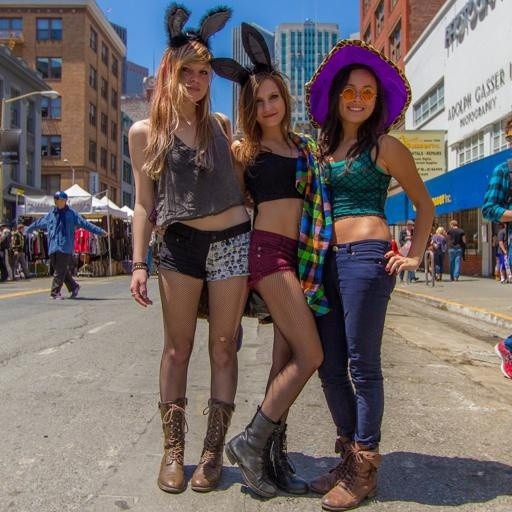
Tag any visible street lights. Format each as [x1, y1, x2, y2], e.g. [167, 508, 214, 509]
[0, 90, 58, 224]
[64, 158, 75, 185]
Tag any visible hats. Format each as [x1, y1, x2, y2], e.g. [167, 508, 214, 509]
[304, 40, 411, 135]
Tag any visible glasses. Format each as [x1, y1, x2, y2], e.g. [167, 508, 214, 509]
[336, 87, 378, 104]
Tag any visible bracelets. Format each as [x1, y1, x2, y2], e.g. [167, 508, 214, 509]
[130, 262, 149, 271]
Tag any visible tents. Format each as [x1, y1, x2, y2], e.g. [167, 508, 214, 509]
[21, 183, 135, 278]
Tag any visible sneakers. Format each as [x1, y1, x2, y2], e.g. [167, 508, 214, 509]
[70, 284, 80, 297]
[494, 340, 512, 378]
[49, 292, 63, 299]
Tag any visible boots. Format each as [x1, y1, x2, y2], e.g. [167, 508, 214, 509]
[191, 398, 236, 493]
[264, 424, 310, 495]
[308, 435, 355, 494]
[157, 397, 188, 493]
[225, 405, 282, 497]
[321, 443, 381, 511]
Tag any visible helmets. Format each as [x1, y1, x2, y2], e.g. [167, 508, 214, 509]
[54, 190, 68, 201]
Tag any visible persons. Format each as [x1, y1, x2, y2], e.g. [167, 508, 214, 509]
[0, 223, 14, 281]
[12, 224, 31, 279]
[498, 221, 510, 282]
[305, 38, 435, 512]
[208, 23, 332, 497]
[26, 191, 108, 299]
[481, 114, 512, 379]
[446, 220, 466, 281]
[399, 220, 420, 281]
[425, 221, 438, 274]
[8, 223, 16, 232]
[131, 4, 253, 494]
[431, 226, 447, 280]
[391, 234, 399, 275]
